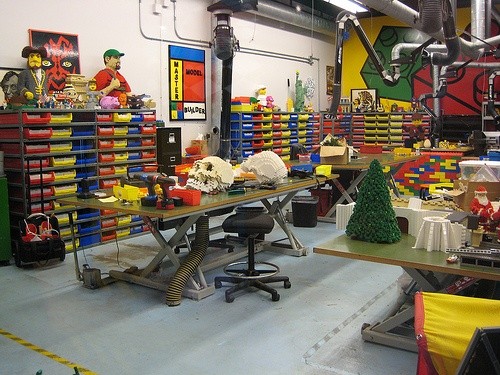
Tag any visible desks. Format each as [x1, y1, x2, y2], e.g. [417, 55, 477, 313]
[285, 153, 420, 224]
[312, 234, 500, 354]
[55, 174, 340, 303]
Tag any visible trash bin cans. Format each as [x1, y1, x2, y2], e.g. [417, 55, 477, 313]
[292, 195, 319, 228]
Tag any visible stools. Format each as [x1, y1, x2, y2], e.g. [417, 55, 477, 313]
[214, 212, 291, 303]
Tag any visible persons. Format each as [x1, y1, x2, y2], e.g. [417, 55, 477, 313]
[354, 99, 361, 112]
[470, 186, 495, 242]
[94, 49, 131, 109]
[403, 113, 425, 140]
[18, 46, 48, 100]
[86, 77, 104, 109]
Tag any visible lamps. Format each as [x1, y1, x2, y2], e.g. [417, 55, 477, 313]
[323, 0, 371, 14]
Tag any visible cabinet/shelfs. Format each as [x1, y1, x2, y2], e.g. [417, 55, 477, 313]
[0, 108, 160, 254]
[351, 113, 433, 148]
[320, 111, 351, 146]
[156, 127, 183, 231]
[221, 111, 321, 160]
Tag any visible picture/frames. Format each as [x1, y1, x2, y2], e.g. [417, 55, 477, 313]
[350, 88, 378, 112]
[28, 29, 81, 94]
[168, 45, 208, 122]
[326, 65, 334, 95]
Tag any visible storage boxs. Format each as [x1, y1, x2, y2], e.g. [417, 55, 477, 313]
[320, 146, 349, 165]
[458, 160, 500, 180]
[453, 180, 500, 213]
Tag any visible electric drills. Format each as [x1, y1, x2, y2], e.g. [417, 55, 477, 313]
[134, 173, 182, 210]
